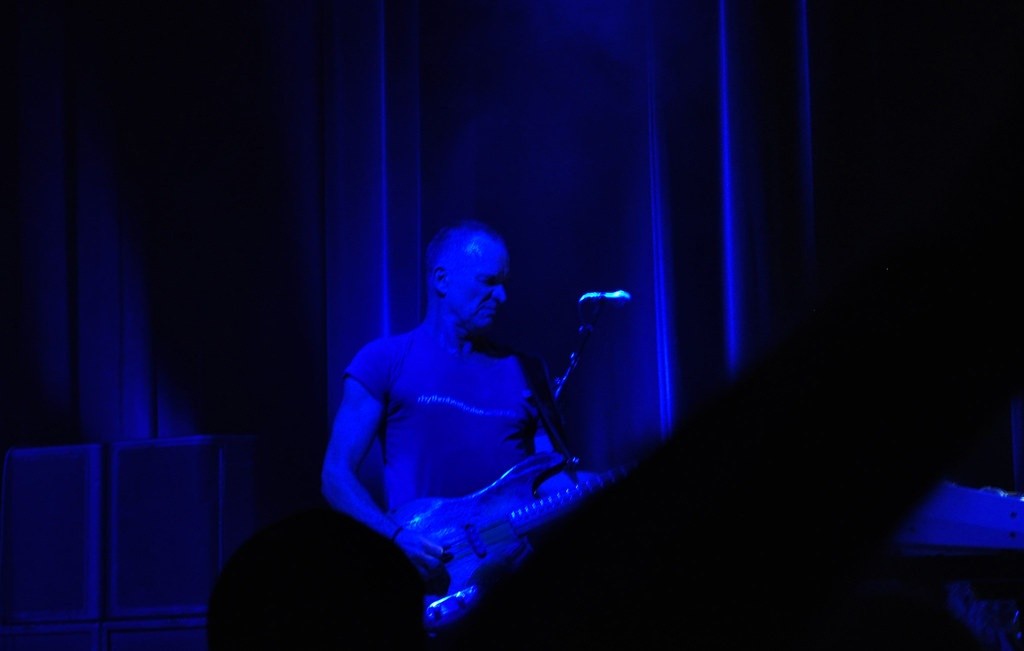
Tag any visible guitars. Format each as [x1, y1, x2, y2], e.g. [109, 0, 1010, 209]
[386, 450, 642, 626]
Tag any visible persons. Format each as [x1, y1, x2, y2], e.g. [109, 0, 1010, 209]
[318, 220, 644, 597]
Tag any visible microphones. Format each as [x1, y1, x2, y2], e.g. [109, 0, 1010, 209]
[581, 289, 631, 304]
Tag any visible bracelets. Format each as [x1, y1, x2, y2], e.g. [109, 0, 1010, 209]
[389, 526, 405, 544]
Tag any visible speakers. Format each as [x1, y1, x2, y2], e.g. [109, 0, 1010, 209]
[1, 436, 255, 651]
